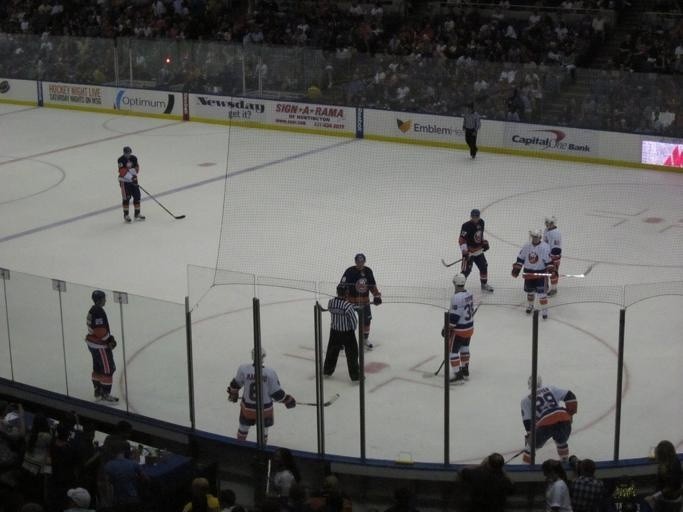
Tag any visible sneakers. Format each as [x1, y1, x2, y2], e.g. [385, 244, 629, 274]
[363, 339, 372, 348]
[135, 214, 145, 219]
[449, 367, 468, 383]
[124, 216, 131, 222]
[542, 309, 547, 318]
[526, 304, 533, 313]
[547, 289, 556, 296]
[481, 283, 494, 292]
[103, 394, 119, 402]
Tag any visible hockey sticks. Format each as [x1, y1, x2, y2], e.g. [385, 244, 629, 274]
[441, 257, 463, 268]
[520, 263, 594, 281]
[138, 184, 186, 219]
[237, 393, 340, 407]
[318, 301, 375, 312]
[423, 300, 483, 380]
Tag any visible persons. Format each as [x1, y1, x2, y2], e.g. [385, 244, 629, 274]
[0, 400, 683, 511]
[324, 284, 365, 381]
[0, 0, 683, 138]
[458, 209, 495, 292]
[519, 374, 578, 464]
[227, 347, 297, 445]
[543, 215, 560, 298]
[441, 273, 474, 383]
[117, 146, 145, 223]
[511, 231, 553, 320]
[338, 253, 382, 349]
[462, 101, 481, 158]
[84, 290, 119, 402]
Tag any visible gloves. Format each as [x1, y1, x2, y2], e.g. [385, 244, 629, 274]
[106, 336, 116, 348]
[374, 297, 382, 305]
[483, 242, 488, 251]
[512, 268, 520, 277]
[286, 396, 296, 409]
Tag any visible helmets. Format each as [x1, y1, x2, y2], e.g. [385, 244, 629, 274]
[529, 229, 541, 239]
[471, 209, 480, 218]
[528, 375, 541, 388]
[454, 274, 466, 285]
[252, 348, 265, 360]
[544, 215, 556, 225]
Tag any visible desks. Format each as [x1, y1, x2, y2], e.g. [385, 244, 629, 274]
[7, 410, 194, 510]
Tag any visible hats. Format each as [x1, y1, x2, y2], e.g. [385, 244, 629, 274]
[67, 488, 91, 508]
